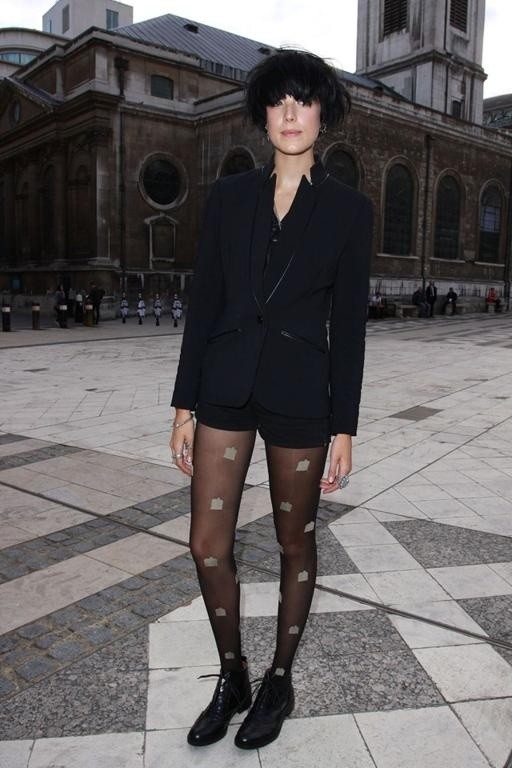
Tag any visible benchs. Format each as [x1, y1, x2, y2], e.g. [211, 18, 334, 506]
[367, 296, 512, 320]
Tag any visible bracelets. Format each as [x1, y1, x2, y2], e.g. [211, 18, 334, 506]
[173, 412, 193, 429]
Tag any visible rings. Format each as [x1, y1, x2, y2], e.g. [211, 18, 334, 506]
[335, 474, 350, 489]
[186, 442, 193, 450]
[172, 455, 176, 458]
[175, 453, 184, 459]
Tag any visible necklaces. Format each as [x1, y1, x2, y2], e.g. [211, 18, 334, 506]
[274, 197, 280, 222]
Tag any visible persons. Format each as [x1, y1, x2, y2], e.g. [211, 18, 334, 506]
[74, 290, 87, 323]
[427, 280, 437, 318]
[169, 49, 376, 751]
[487, 287, 501, 312]
[55, 283, 67, 327]
[371, 291, 385, 321]
[411, 287, 429, 318]
[87, 280, 102, 325]
[440, 287, 458, 316]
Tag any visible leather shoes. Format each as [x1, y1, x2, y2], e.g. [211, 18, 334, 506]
[232, 664, 298, 752]
[184, 654, 252, 748]
